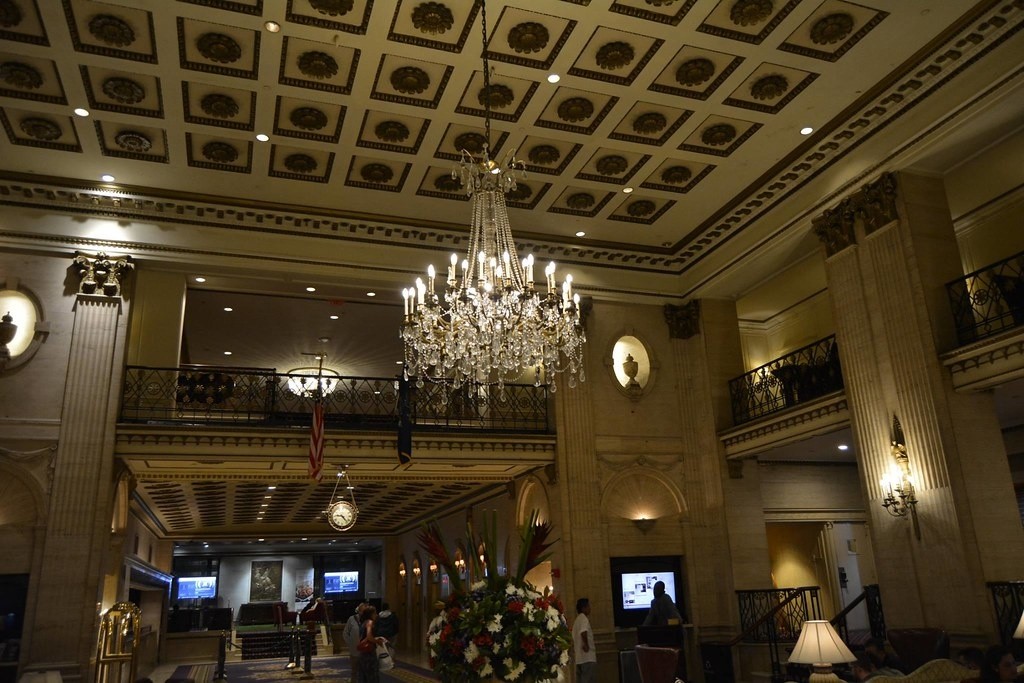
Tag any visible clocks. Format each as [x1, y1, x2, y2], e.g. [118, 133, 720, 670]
[328, 476, 359, 531]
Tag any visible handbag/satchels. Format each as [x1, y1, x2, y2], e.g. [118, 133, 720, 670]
[357, 637, 373, 654]
[376, 643, 395, 673]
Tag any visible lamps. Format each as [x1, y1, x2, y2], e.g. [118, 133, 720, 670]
[286, 367, 341, 398]
[788, 619, 858, 683]
[633, 519, 656, 534]
[396, 0, 587, 404]
[878, 463, 917, 518]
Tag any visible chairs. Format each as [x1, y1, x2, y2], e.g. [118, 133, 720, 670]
[635, 644, 678, 683]
[886, 628, 949, 673]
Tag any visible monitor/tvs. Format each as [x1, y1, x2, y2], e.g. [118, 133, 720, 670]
[178, 577, 216, 599]
[325, 571, 358, 594]
[622, 572, 676, 610]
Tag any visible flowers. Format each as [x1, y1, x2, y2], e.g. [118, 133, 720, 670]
[416, 510, 573, 683]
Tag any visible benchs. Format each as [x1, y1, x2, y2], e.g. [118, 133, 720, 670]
[865, 661, 1024, 683]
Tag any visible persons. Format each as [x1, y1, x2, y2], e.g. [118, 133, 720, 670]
[167, 603, 180, 623]
[840, 637, 1024, 683]
[425, 600, 445, 635]
[299, 597, 321, 626]
[643, 581, 683, 626]
[572, 598, 597, 683]
[343, 602, 400, 683]
